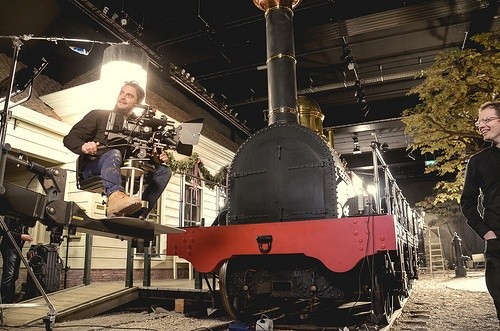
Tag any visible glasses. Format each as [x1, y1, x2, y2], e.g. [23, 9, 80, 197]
[475, 116, 500, 126]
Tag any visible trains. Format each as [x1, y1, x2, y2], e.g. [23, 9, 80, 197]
[166, 0, 419, 330]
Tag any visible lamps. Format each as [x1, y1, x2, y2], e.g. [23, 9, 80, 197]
[0, 35, 150, 331]
[370, 141, 389, 153]
[353, 139, 361, 154]
[356, 89, 369, 117]
[340, 46, 353, 67]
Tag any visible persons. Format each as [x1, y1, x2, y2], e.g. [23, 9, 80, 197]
[0, 214, 33, 302]
[460, 101, 500, 321]
[62, 80, 172, 220]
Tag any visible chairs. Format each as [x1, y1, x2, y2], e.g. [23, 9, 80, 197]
[74, 157, 112, 193]
[472, 253, 486, 270]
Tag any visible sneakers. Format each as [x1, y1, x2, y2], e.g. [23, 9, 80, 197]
[107, 190, 142, 217]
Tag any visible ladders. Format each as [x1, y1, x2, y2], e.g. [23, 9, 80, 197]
[427, 226, 448, 277]
